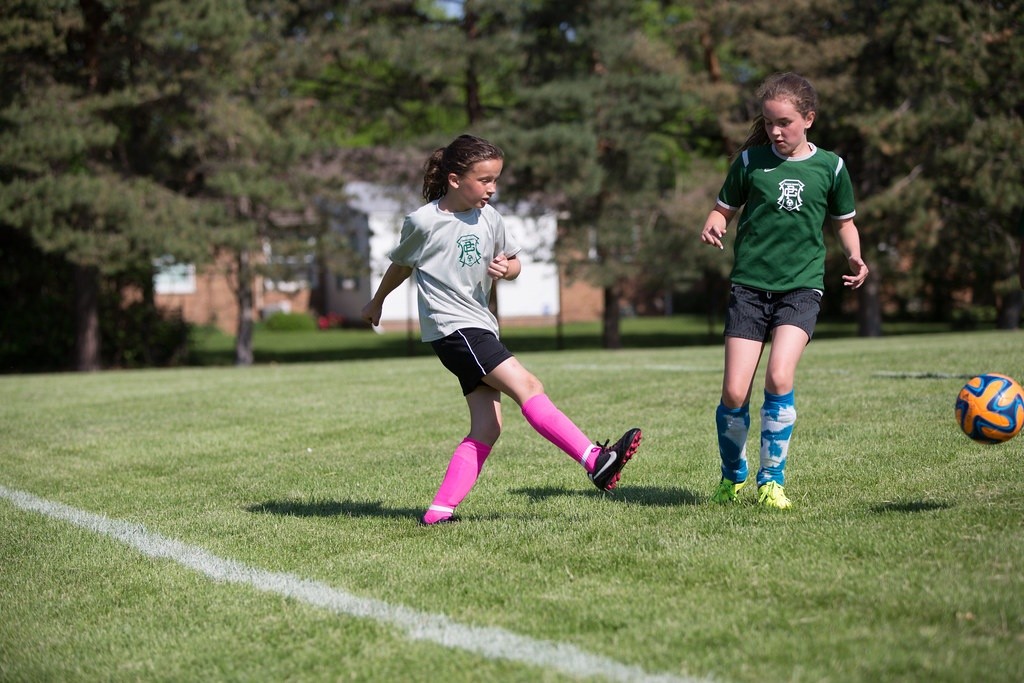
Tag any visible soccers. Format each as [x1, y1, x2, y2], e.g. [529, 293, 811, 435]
[954, 371, 1024, 446]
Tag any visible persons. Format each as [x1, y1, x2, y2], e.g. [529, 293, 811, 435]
[363, 134, 643, 525]
[700, 73, 870, 511]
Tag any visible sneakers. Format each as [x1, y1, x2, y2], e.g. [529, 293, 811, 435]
[757, 480, 792, 510]
[587, 428, 642, 491]
[708, 476, 748, 504]
[419, 516, 461, 527]
